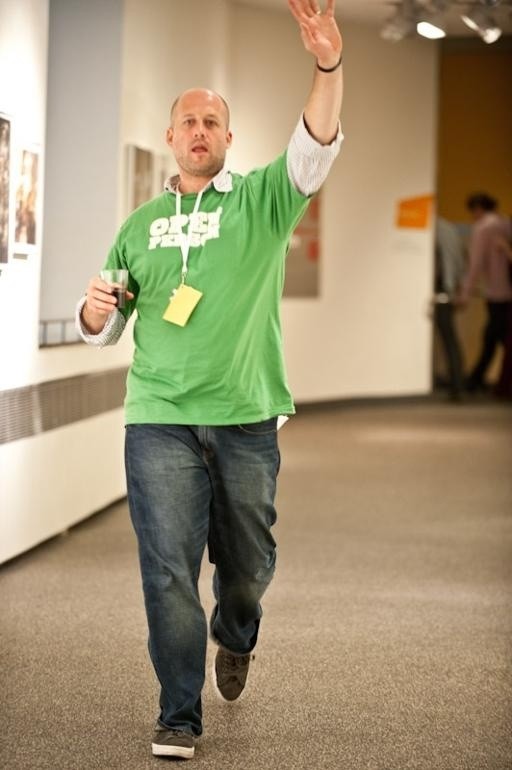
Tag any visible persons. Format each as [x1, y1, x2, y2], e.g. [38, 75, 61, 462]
[74, 0, 347, 757]
[434, 212, 475, 402]
[458, 193, 512, 397]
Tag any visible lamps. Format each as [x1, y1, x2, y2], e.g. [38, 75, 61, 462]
[380, 0, 506, 46]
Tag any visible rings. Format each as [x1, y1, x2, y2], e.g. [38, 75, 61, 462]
[316, 10, 321, 15]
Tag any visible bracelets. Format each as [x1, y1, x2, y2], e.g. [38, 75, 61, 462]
[313, 53, 343, 72]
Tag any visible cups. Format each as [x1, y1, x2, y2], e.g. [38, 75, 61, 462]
[99, 269, 129, 309]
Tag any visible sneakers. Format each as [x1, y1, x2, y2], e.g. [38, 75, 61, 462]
[211, 646, 252, 705]
[150, 724, 197, 761]
